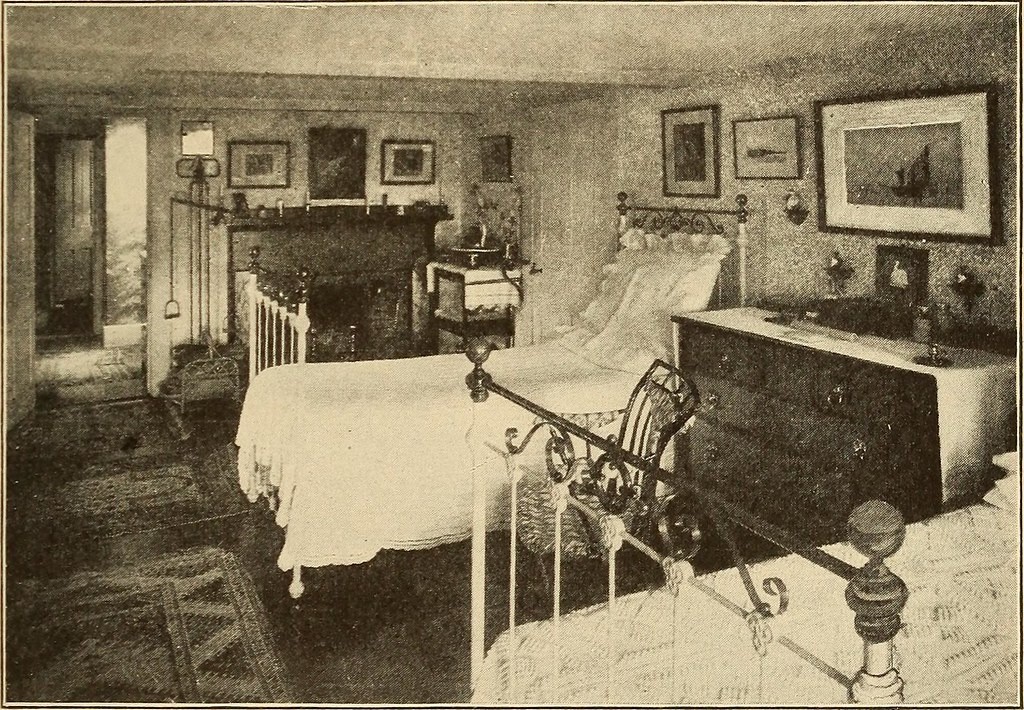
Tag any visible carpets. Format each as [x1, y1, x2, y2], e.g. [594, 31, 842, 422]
[5, 446, 250, 544]
[7, 549, 296, 705]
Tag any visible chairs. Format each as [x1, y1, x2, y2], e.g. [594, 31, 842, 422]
[503, 355, 705, 610]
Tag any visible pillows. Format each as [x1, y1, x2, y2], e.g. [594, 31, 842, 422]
[557, 226, 732, 386]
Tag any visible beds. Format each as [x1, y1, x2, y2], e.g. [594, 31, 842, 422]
[232, 340, 681, 603]
[464, 495, 1020, 705]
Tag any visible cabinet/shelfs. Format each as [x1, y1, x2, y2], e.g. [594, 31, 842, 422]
[673, 305, 1018, 570]
[427, 261, 521, 355]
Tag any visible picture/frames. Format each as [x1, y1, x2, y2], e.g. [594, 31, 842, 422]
[180, 119, 216, 157]
[815, 86, 1002, 244]
[379, 138, 436, 185]
[731, 116, 801, 181]
[876, 245, 928, 305]
[225, 138, 292, 189]
[661, 106, 721, 197]
[480, 134, 515, 184]
